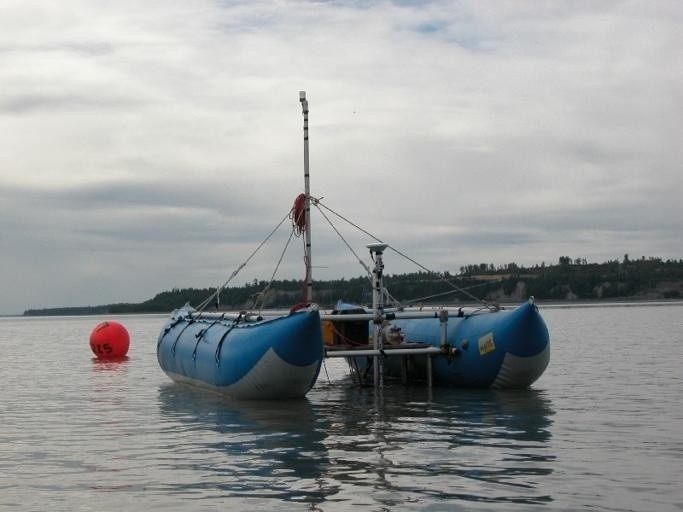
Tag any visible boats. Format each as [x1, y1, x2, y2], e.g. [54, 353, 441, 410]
[153, 90, 551, 399]
